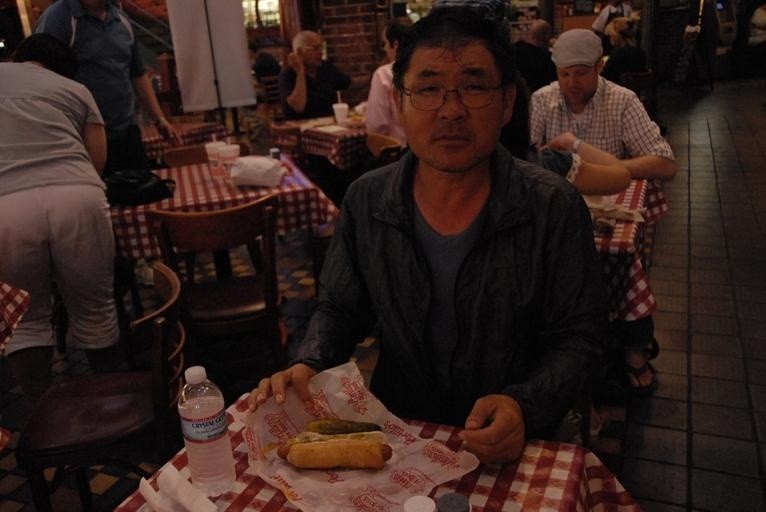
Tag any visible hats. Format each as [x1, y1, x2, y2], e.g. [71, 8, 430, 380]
[551, 28, 603, 69]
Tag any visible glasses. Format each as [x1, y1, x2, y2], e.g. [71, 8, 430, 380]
[303, 43, 324, 52]
[397, 78, 508, 113]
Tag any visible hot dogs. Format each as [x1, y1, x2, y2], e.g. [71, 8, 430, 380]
[276, 430, 392, 470]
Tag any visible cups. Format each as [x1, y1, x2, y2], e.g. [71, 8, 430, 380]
[332, 104, 349, 124]
[205, 141, 240, 181]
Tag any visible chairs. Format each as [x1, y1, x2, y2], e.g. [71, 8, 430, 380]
[13, 262, 185, 510]
[139, 188, 286, 389]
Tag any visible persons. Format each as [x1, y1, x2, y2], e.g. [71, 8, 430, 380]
[356, 20, 410, 178]
[247, 28, 281, 105]
[0, 32, 121, 407]
[499, 75, 632, 194]
[278, 31, 353, 207]
[248, 2, 606, 462]
[526, 28, 677, 396]
[478, 1, 766, 91]
[33, 1, 185, 374]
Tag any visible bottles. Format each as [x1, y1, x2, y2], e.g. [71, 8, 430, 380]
[176, 366, 236, 492]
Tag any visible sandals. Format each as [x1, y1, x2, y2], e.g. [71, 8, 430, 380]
[621, 359, 658, 394]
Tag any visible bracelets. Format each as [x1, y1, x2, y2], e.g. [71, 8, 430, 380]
[154, 117, 166, 127]
[572, 138, 582, 150]
